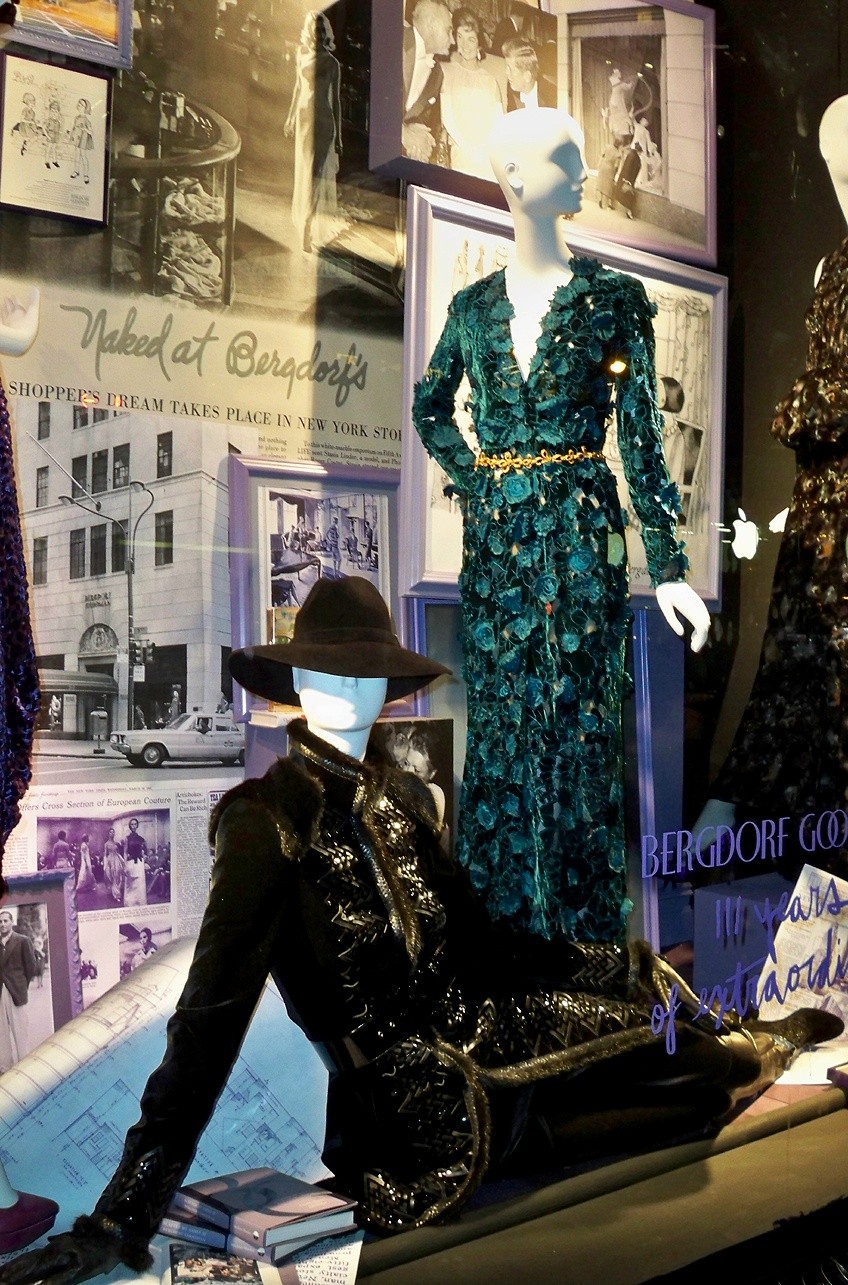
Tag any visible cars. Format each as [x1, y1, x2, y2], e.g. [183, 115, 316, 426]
[111, 711, 245, 768]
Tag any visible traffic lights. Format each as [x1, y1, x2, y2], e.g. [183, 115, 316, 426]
[132, 640, 143, 665]
[146, 641, 156, 665]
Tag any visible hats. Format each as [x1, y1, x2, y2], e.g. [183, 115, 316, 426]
[227, 576, 451, 706]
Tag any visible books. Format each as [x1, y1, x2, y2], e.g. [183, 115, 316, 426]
[157, 1166, 360, 1267]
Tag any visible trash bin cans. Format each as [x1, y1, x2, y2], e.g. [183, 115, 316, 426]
[90, 711, 107, 735]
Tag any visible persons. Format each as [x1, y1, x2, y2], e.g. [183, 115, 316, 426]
[285, 12, 344, 251]
[412, 108, 710, 941]
[135, 689, 230, 735]
[400, 1, 666, 218]
[1, 818, 173, 1079]
[0, 575, 843, 1285]
[272, 515, 378, 608]
[649, 94, 848, 968]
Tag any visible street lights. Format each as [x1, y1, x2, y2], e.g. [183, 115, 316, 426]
[58, 481, 154, 729]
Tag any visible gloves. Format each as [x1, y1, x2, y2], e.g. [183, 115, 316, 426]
[0, 1214, 125, 1285]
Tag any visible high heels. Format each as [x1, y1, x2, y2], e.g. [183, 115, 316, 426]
[738, 1006, 846, 1049]
